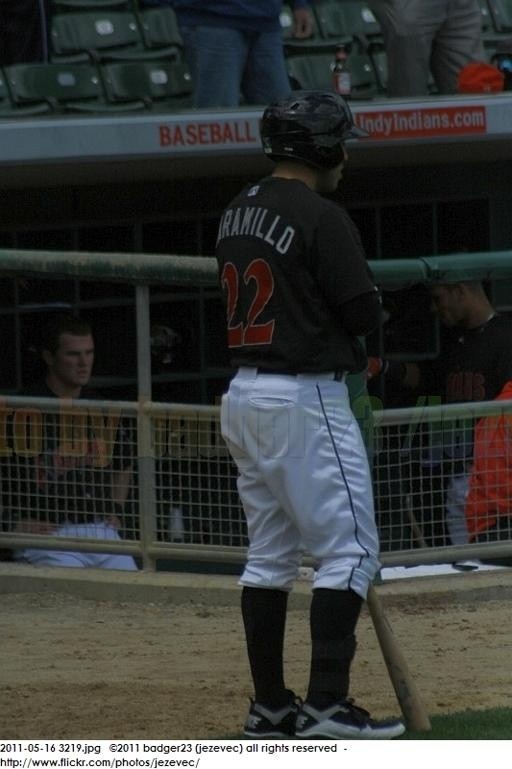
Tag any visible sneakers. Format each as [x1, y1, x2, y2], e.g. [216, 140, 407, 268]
[293, 700, 408, 738]
[242, 690, 294, 738]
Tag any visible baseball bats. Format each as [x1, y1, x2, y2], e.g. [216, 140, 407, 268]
[365, 584, 432, 733]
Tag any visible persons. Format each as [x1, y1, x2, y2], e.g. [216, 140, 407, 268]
[215, 88, 406, 739]
[0, 274, 511, 570]
[364, 1, 483, 98]
[173, 1, 319, 109]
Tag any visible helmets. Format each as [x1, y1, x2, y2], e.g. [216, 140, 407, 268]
[258, 87, 371, 172]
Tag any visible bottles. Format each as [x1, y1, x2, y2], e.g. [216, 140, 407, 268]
[331, 44, 355, 100]
